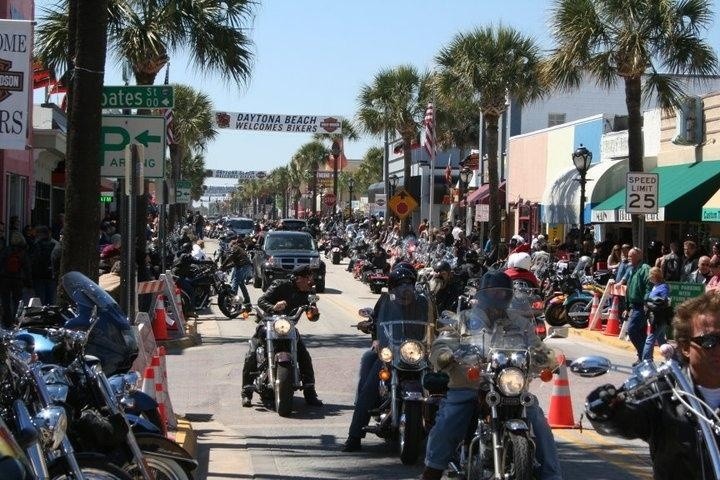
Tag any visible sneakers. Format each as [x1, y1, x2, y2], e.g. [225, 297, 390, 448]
[241, 398, 250, 407]
[340, 437, 361, 452]
[305, 396, 322, 406]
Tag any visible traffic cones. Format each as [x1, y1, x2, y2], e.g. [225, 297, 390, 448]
[135, 345, 170, 429]
[543, 351, 581, 431]
[587, 293, 606, 332]
[600, 296, 621, 336]
[156, 291, 190, 337]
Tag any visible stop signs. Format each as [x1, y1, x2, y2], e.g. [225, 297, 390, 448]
[323, 193, 337, 207]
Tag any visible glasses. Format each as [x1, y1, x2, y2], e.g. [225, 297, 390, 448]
[690, 333, 720, 352]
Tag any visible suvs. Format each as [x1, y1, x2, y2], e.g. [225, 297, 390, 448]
[251, 229, 327, 293]
[220, 215, 257, 239]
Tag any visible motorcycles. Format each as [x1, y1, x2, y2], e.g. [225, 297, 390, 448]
[237, 293, 321, 415]
[567, 340, 720, 480]
[0, 250, 244, 480]
[350, 292, 474, 464]
[311, 210, 640, 349]
[428, 311, 574, 480]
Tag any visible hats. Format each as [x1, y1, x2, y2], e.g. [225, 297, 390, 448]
[292, 264, 313, 275]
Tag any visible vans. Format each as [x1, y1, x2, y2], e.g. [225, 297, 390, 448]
[277, 218, 310, 231]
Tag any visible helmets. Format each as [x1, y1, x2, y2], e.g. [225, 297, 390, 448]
[480, 272, 513, 306]
[389, 262, 417, 288]
[466, 250, 478, 263]
[435, 261, 451, 272]
[182, 243, 192, 253]
[508, 252, 531, 271]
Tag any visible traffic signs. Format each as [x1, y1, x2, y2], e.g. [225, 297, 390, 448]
[99, 87, 176, 111]
[623, 168, 670, 224]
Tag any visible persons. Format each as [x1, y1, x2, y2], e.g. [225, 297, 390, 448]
[100, 211, 324, 409]
[1, 211, 65, 327]
[305, 211, 720, 479]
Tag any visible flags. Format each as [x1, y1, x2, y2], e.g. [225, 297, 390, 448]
[420, 102, 437, 159]
[160, 69, 174, 146]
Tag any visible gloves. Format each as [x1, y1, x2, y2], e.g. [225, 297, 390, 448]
[585, 383, 618, 415]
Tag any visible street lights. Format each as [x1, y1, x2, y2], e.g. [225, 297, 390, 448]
[570, 142, 595, 252]
[388, 173, 399, 229]
[457, 164, 475, 200]
[347, 176, 354, 216]
